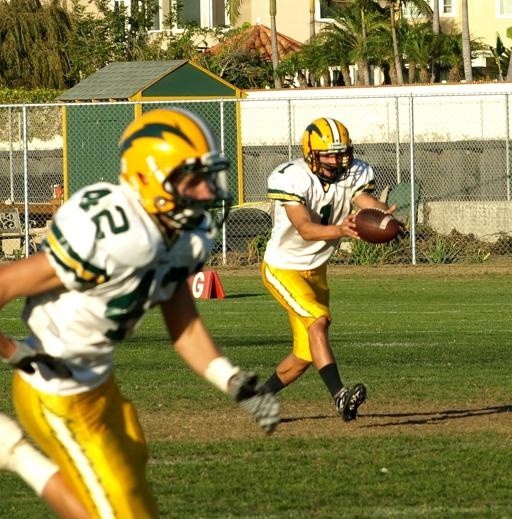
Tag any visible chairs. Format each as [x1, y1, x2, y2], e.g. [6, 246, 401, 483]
[0, 208, 36, 258]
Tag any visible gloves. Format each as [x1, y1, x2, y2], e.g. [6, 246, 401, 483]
[225, 369, 281, 436]
[3, 340, 74, 381]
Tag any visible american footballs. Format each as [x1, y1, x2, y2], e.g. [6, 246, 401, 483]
[350, 208, 398, 242]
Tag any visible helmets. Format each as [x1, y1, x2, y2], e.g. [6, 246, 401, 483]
[301, 117, 355, 183]
[118, 105, 233, 233]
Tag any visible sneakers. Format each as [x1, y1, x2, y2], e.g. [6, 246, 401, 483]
[337, 381, 366, 423]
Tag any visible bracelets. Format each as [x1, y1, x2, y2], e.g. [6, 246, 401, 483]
[203, 355, 242, 400]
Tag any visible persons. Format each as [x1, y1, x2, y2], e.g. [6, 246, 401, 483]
[261, 116, 413, 422]
[0, 104, 280, 518]
